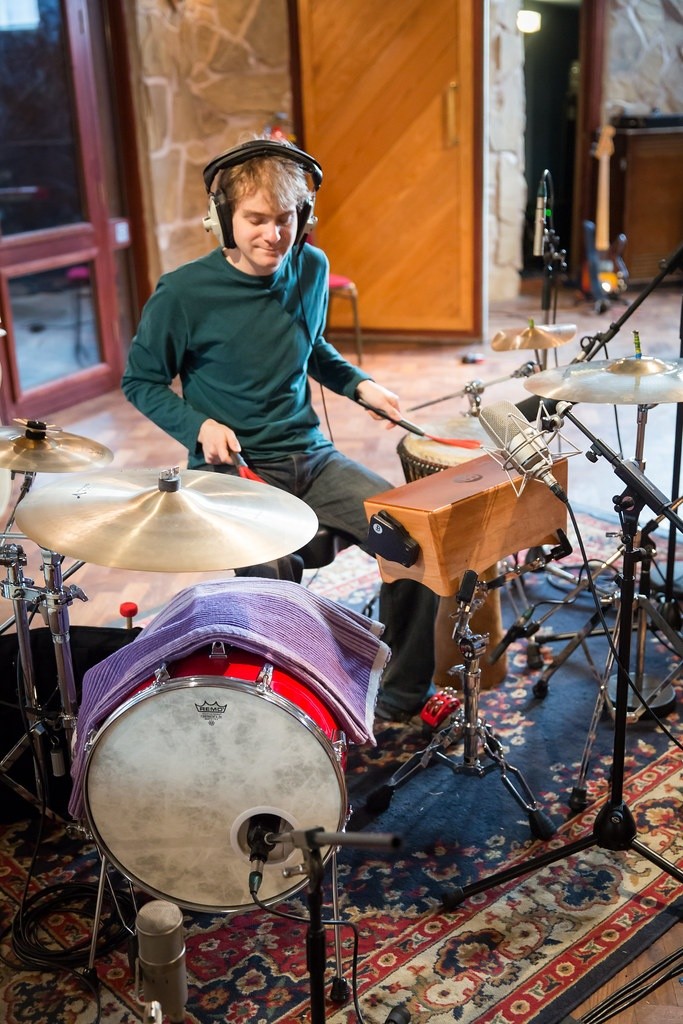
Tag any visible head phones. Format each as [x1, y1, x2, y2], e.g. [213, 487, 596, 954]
[201, 140, 324, 248]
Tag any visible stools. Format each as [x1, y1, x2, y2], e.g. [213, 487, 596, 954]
[69, 267, 94, 355]
[323, 274, 362, 368]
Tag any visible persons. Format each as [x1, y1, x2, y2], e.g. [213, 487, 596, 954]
[121, 135, 456, 731]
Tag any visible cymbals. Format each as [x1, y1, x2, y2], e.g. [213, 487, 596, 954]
[490, 316, 578, 352]
[0, 417, 115, 473]
[523, 330, 683, 405]
[15, 466, 319, 573]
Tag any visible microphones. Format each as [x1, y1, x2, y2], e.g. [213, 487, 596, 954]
[533, 181, 548, 257]
[135, 899, 188, 1024]
[247, 813, 281, 895]
[480, 399, 569, 505]
[488, 604, 534, 665]
[571, 339, 596, 366]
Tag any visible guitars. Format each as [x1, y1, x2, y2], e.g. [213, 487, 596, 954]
[579, 124, 629, 301]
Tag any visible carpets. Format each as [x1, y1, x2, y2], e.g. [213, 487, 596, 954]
[0, 496, 682, 1024]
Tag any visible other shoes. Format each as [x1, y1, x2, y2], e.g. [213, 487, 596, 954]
[378, 705, 415, 727]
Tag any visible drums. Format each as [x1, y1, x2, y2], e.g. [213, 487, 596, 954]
[395, 413, 511, 690]
[81, 645, 352, 914]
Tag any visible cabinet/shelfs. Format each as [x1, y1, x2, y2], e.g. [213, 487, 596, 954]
[609, 127, 683, 286]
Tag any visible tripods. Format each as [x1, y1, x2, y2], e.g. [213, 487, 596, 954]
[0, 547, 95, 845]
[369, 247, 683, 910]
